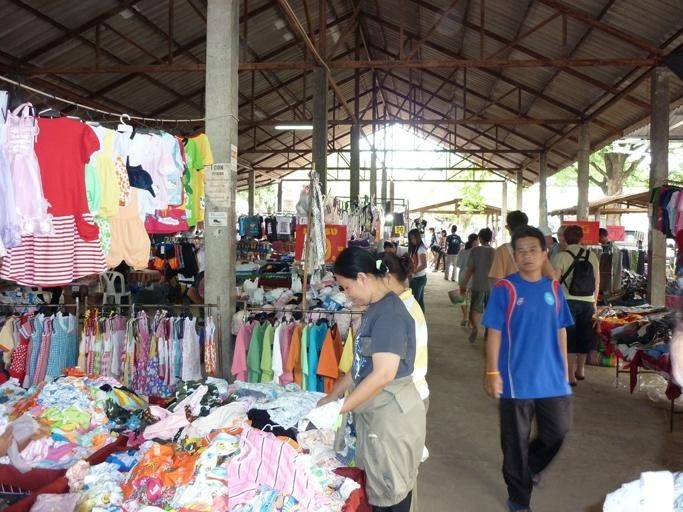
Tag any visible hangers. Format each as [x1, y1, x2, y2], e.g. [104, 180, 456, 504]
[244, 308, 353, 332]
[0, 78, 203, 138]
[238, 210, 295, 219]
[85, 306, 214, 323]
[149, 235, 199, 247]
[0, 305, 75, 320]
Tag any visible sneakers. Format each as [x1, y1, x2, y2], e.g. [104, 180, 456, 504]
[527, 468, 541, 485]
[508, 498, 533, 512]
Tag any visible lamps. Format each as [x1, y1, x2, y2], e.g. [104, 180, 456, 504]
[275, 125, 315, 130]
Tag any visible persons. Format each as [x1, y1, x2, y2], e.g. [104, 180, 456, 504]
[487, 209, 556, 288]
[428, 228, 448, 273]
[553, 224, 600, 387]
[460, 228, 495, 342]
[481, 228, 576, 512]
[383, 241, 408, 259]
[444, 224, 459, 282]
[455, 234, 479, 326]
[600, 228, 622, 253]
[380, 251, 430, 405]
[315, 246, 428, 511]
[406, 229, 428, 314]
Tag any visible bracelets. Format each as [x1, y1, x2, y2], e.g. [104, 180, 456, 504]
[484, 371, 503, 375]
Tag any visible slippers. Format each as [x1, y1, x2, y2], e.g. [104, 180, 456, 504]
[575, 374, 584, 380]
[569, 379, 576, 385]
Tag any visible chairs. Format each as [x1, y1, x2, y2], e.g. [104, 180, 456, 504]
[99, 270, 132, 315]
[23, 285, 64, 305]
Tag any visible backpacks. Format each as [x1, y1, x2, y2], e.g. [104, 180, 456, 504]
[560, 249, 596, 298]
